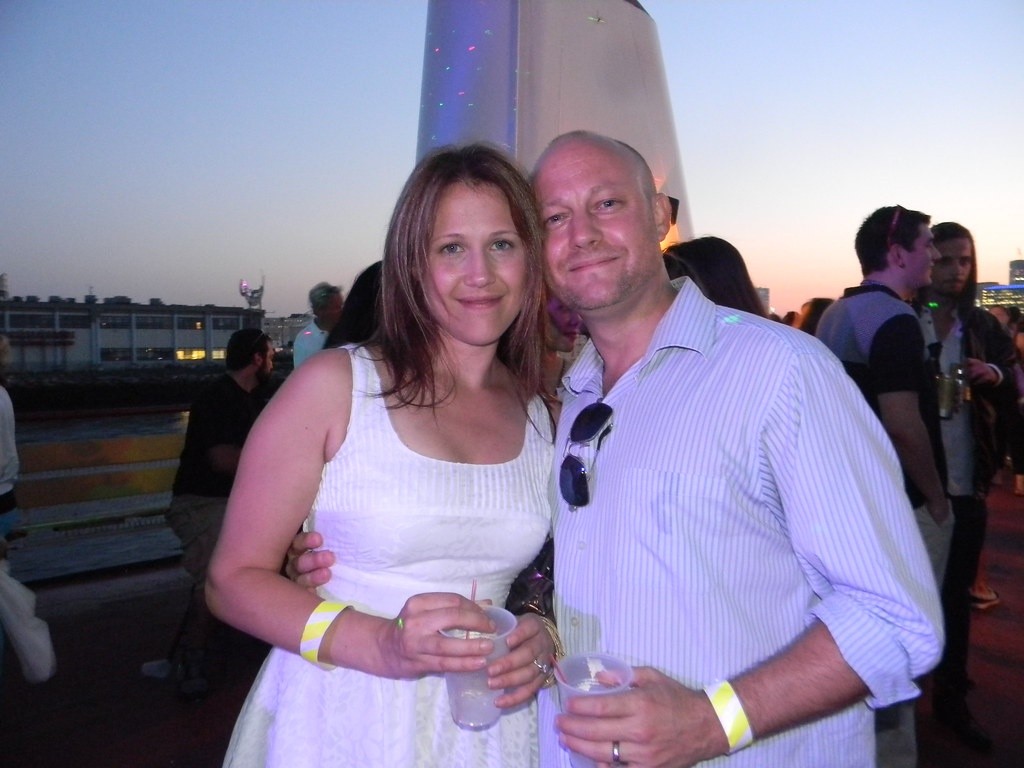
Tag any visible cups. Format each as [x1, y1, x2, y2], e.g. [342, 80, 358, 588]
[554, 653, 634, 768]
[437, 605, 518, 730]
[936, 374, 956, 419]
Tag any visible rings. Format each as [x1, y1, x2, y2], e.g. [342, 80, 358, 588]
[613, 740, 620, 767]
[533, 656, 550, 674]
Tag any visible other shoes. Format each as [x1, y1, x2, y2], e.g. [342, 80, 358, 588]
[936, 704, 994, 745]
[176, 660, 208, 705]
[971, 586, 998, 608]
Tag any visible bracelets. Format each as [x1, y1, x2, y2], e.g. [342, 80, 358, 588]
[300, 600, 356, 664]
[536, 613, 564, 688]
[702, 679, 753, 754]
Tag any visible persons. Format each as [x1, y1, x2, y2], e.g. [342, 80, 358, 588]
[813, 208, 1024, 768]
[0, 336, 53, 688]
[166, 327, 274, 706]
[204, 144, 564, 768]
[284, 131, 945, 768]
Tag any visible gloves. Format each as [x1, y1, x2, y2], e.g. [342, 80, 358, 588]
[0, 486, 30, 549]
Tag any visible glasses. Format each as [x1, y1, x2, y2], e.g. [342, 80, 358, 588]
[252, 328, 267, 347]
[887, 203, 909, 243]
[559, 403, 614, 513]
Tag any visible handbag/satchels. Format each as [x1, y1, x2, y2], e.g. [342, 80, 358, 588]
[504, 537, 556, 621]
